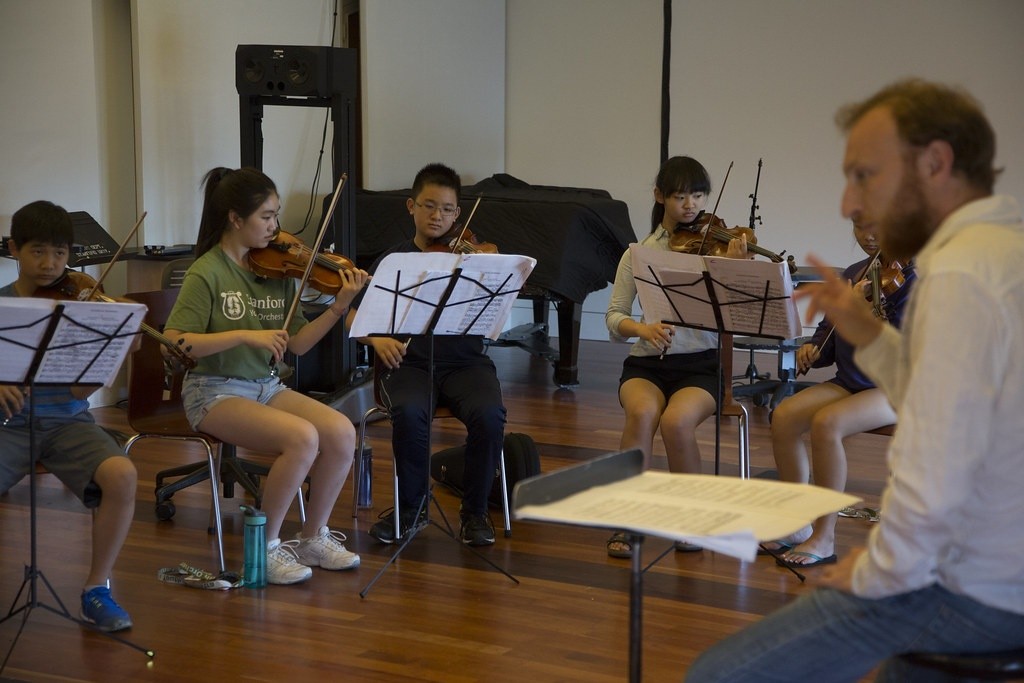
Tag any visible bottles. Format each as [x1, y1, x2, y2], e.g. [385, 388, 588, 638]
[238, 503, 268, 590]
[352, 436, 374, 509]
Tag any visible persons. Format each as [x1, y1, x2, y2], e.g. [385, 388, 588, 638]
[605, 154, 755, 556]
[684, 78, 1024, 683]
[345, 163, 502, 543]
[0, 199, 144, 632]
[756, 224, 916, 567]
[160, 167, 361, 584]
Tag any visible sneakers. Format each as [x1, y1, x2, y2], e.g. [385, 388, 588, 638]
[288, 526, 361, 572]
[79, 586, 133, 633]
[367, 506, 429, 545]
[241, 537, 313, 585]
[459, 508, 497, 548]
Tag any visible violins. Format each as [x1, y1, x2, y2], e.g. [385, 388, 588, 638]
[422, 223, 500, 255]
[251, 228, 372, 296]
[667, 211, 799, 275]
[851, 256, 912, 321]
[36, 270, 200, 370]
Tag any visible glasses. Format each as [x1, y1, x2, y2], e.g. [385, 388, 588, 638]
[413, 198, 457, 216]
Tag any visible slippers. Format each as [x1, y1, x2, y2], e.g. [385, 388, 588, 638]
[755, 538, 792, 555]
[775, 545, 837, 569]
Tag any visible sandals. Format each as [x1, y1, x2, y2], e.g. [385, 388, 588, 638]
[673, 540, 703, 553]
[605, 529, 633, 559]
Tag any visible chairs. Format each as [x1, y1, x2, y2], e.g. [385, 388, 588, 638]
[34, 258, 1024, 683]
